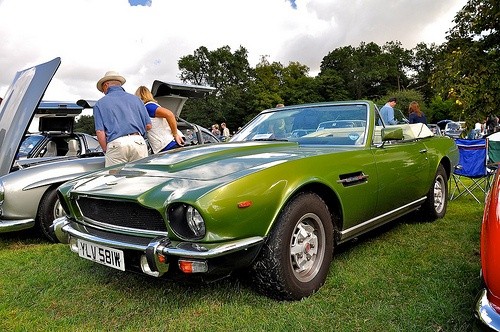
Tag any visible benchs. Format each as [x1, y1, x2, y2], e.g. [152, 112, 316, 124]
[313, 123, 434, 144]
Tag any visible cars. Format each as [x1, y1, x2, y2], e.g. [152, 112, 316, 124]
[467, 163, 500, 332]
[289, 120, 468, 144]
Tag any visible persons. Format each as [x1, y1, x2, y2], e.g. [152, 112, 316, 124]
[483, 114, 499, 132]
[409, 101, 427, 126]
[93, 71, 152, 168]
[378, 98, 397, 126]
[221, 123, 229, 142]
[135, 85, 186, 154]
[269, 104, 285, 138]
[211, 124, 221, 136]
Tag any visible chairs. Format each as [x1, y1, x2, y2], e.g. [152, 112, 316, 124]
[65, 139, 79, 157]
[451, 137, 500, 205]
[42, 141, 58, 157]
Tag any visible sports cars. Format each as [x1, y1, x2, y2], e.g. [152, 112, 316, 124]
[0, 57, 226, 244]
[48, 99, 461, 302]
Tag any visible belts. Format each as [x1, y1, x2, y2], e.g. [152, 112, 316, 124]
[124, 132, 138, 136]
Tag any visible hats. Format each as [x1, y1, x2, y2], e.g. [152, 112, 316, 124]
[96, 71, 126, 93]
